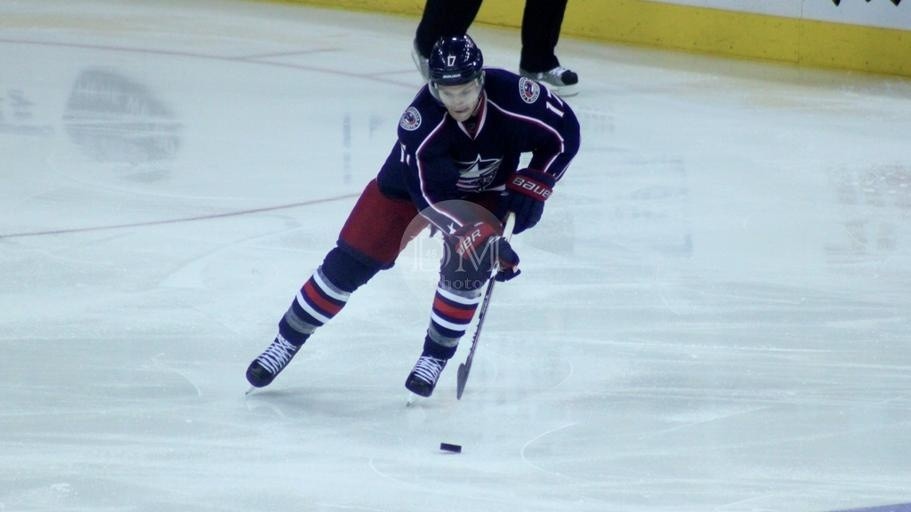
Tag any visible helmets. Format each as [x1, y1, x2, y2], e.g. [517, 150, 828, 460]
[429, 33, 483, 86]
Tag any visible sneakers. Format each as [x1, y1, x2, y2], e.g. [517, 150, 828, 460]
[521, 67, 577, 84]
[247, 333, 301, 388]
[405, 352, 447, 396]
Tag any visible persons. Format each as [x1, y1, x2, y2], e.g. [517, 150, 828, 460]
[245, 32, 581, 398]
[415, 0, 578, 86]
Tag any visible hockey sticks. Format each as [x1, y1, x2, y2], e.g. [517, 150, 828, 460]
[457, 212, 517, 397]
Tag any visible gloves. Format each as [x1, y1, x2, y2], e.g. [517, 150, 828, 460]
[492, 168, 555, 234]
[452, 220, 520, 282]
